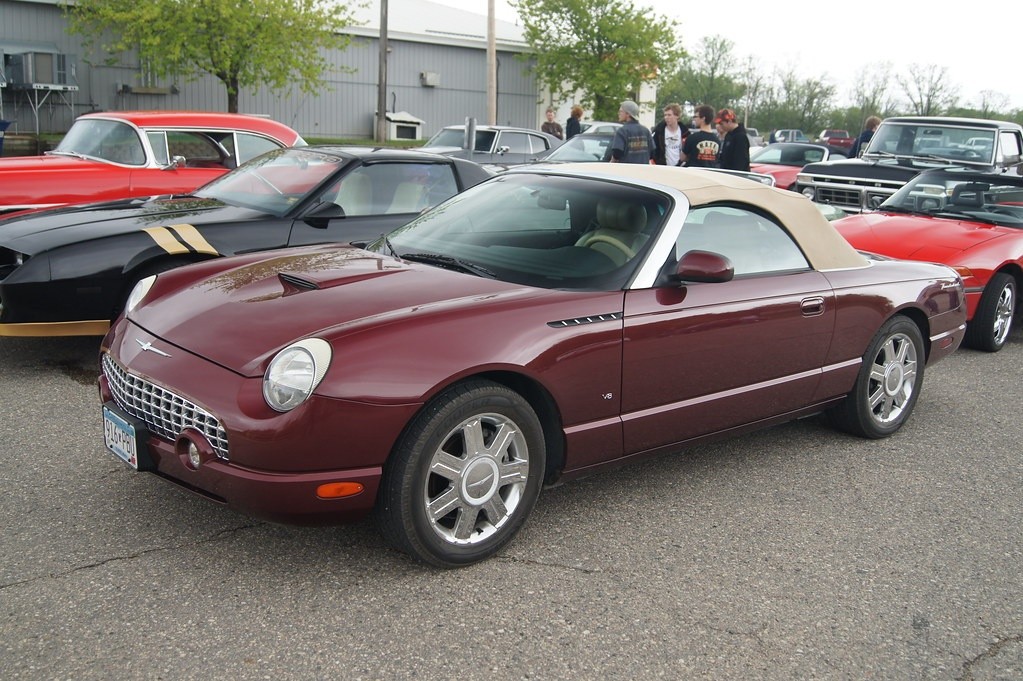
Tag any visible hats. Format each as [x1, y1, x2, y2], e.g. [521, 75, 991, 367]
[620, 101, 639, 122]
[714, 109, 735, 123]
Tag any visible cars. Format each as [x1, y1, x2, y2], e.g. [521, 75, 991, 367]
[407, 124, 599, 178]
[0, 110, 343, 282]
[90, 160, 969, 571]
[496, 133, 616, 175]
[828, 166, 1023, 353]
[1, 143, 574, 338]
[577, 113, 859, 192]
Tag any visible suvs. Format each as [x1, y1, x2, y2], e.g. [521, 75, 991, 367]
[795, 115, 1023, 221]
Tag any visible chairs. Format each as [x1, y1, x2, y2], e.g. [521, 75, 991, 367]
[941, 185, 986, 210]
[577, 199, 649, 265]
[336, 174, 428, 218]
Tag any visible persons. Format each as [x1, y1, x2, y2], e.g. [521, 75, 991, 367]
[609, 101, 656, 164]
[713, 107, 751, 178]
[769, 127, 777, 142]
[847, 116, 881, 157]
[680, 106, 721, 168]
[542, 109, 563, 141]
[649, 103, 691, 167]
[566, 106, 583, 140]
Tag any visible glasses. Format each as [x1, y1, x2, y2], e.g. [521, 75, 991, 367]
[693, 115, 703, 120]
